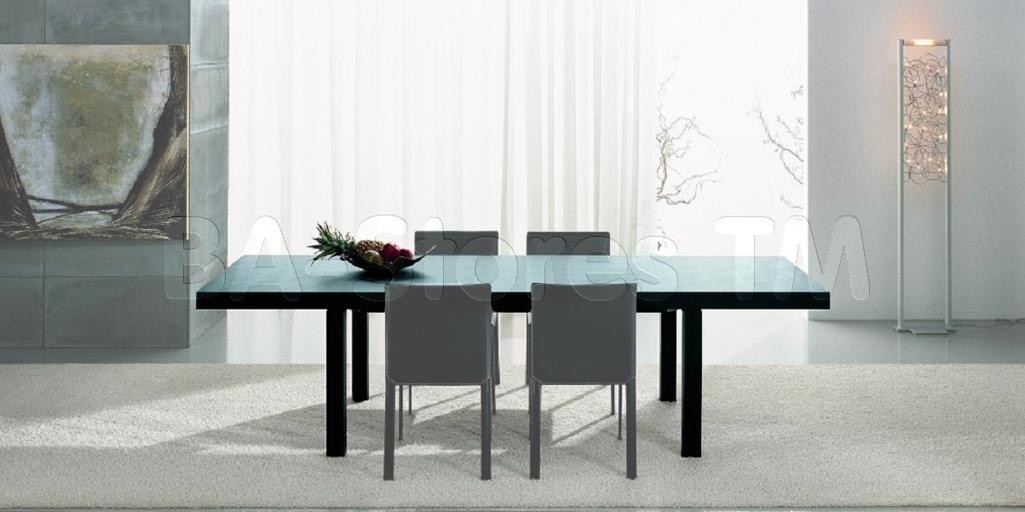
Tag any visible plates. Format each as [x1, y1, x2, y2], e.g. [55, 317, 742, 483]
[347, 244, 438, 275]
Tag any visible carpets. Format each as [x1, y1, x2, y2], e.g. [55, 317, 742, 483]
[0, 361, 1024, 507]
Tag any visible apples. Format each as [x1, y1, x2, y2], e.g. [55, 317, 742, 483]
[400, 249, 414, 257]
[382, 243, 400, 260]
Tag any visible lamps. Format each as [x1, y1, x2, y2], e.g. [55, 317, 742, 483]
[894, 38, 957, 336]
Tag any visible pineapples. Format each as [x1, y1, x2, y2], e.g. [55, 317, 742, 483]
[306, 221, 384, 269]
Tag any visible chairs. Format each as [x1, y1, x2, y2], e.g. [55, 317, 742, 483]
[415, 230, 499, 255]
[527, 282, 637, 479]
[385, 282, 497, 478]
[526, 231, 610, 255]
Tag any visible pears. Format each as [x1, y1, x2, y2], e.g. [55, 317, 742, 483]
[364, 250, 382, 265]
